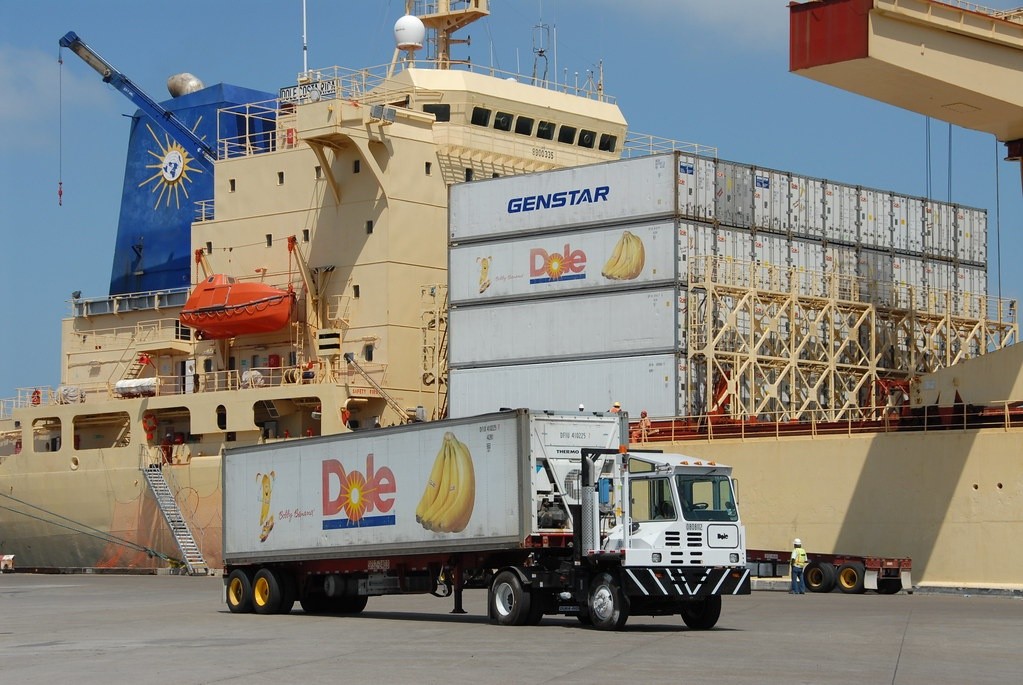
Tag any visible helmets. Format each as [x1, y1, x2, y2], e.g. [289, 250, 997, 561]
[793, 538, 801, 544]
[613, 402, 621, 406]
[579, 403, 585, 408]
[641, 410, 648, 416]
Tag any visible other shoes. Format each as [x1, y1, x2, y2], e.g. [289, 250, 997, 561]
[799, 592, 805, 594]
[789, 591, 798, 594]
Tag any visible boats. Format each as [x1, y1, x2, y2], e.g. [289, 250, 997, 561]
[179, 273, 297, 340]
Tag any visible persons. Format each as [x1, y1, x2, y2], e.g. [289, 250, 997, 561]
[607, 402, 622, 412]
[161, 433, 172, 464]
[630, 411, 650, 443]
[791, 539, 808, 594]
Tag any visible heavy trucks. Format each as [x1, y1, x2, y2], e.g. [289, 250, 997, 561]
[223, 405, 751, 634]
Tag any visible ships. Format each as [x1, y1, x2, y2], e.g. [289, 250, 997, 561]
[1, 0, 1023, 594]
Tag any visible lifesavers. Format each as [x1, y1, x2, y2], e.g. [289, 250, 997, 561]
[32, 390, 41, 405]
[144, 414, 158, 431]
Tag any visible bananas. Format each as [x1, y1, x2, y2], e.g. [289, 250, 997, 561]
[417, 431, 475, 532]
[602, 231, 645, 281]
